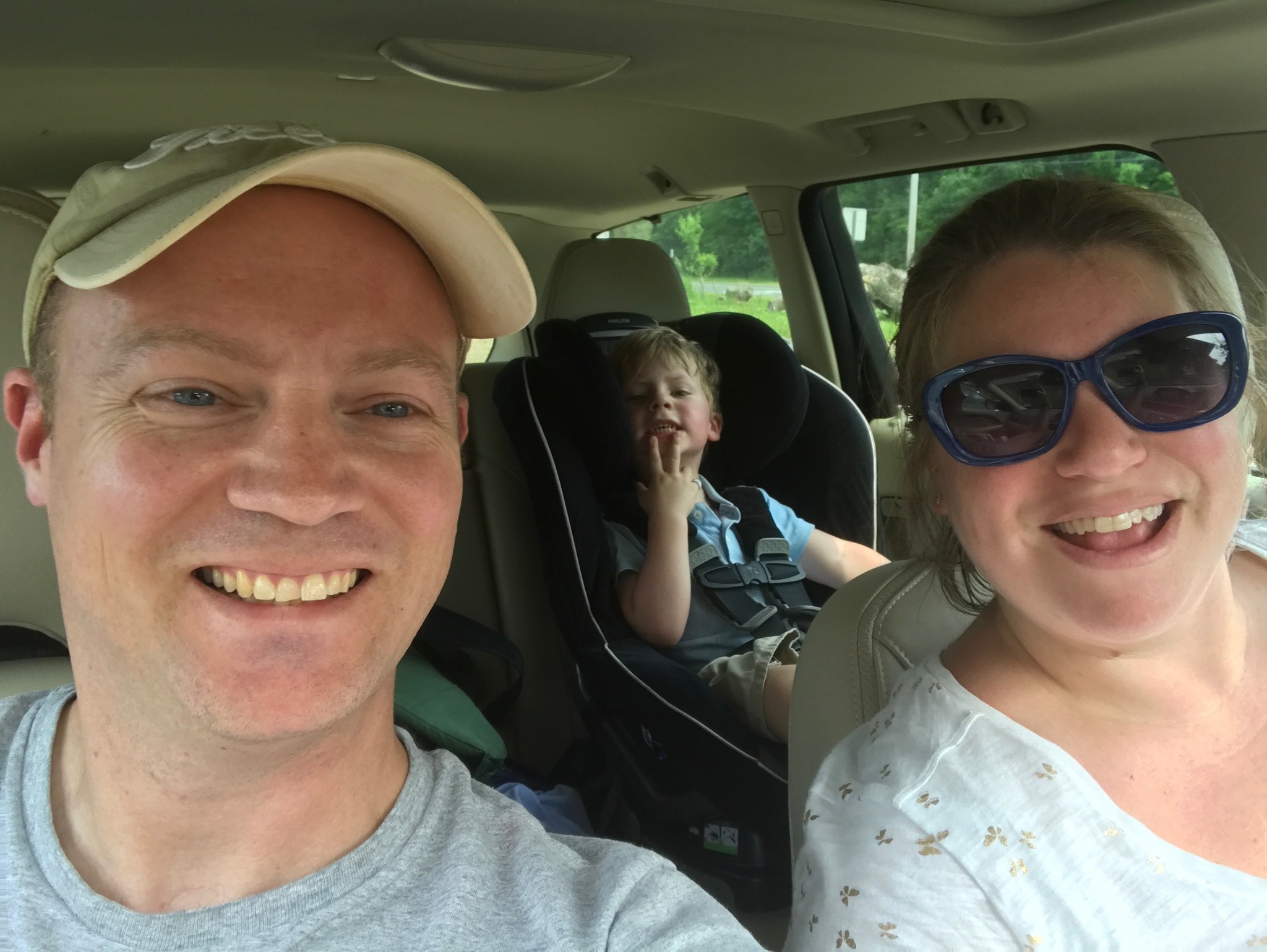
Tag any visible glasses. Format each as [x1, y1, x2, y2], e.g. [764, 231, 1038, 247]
[913, 311, 1250, 467]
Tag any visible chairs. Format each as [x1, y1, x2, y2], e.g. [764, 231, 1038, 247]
[0, 184, 1267, 849]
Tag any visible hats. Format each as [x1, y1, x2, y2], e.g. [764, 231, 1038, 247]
[22, 122, 537, 386]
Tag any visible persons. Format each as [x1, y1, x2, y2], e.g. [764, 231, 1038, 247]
[1, 115, 767, 952]
[792, 176, 1267, 952]
[585, 326, 893, 759]
